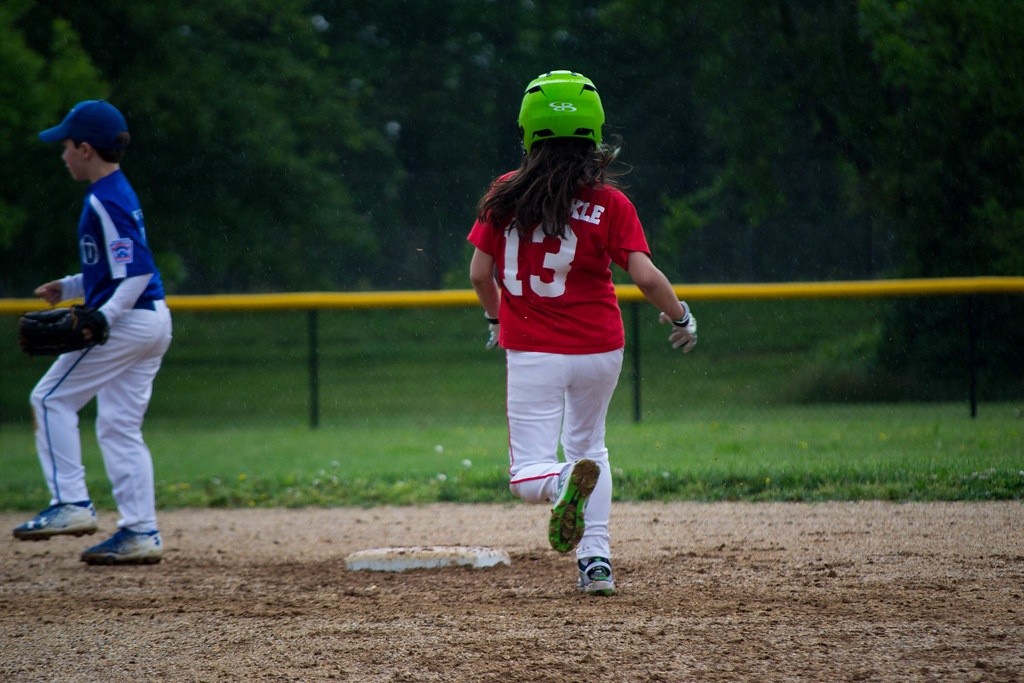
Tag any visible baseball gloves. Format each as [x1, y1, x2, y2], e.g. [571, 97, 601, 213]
[18, 302, 111, 359]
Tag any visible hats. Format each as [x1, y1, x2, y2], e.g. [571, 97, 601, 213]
[38, 100, 129, 151]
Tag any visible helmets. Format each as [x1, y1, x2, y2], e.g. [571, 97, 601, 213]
[518, 70, 605, 156]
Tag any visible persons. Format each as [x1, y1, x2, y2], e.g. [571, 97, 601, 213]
[12, 100, 174, 566]
[466, 69, 699, 597]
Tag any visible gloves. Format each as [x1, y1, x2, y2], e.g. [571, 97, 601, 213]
[659, 301, 697, 353]
[485, 311, 504, 352]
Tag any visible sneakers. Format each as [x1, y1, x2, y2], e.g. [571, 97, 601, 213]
[548, 458, 600, 553]
[577, 556, 617, 596]
[13, 500, 97, 541]
[82, 527, 162, 564]
